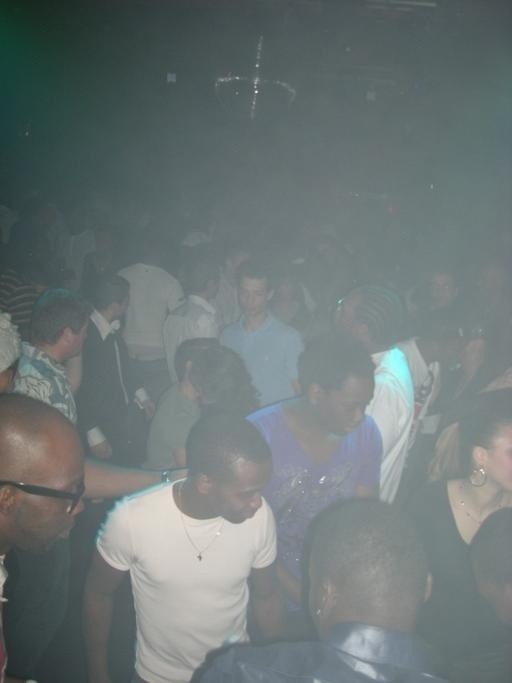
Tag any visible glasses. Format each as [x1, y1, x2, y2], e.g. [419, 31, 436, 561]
[0, 480, 85, 515]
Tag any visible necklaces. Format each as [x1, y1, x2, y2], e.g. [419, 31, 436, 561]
[176, 482, 228, 563]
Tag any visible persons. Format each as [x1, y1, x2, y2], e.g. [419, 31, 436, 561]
[191, 347, 263, 414]
[0, 312, 21, 394]
[80, 413, 286, 683]
[422, 263, 460, 297]
[402, 389, 511, 661]
[12, 288, 93, 427]
[368, 292, 491, 504]
[149, 337, 216, 469]
[164, 254, 222, 378]
[194, 499, 461, 683]
[221, 259, 304, 406]
[77, 275, 155, 462]
[411, 389, 512, 574]
[1, 392, 85, 682]
[471, 509, 512, 628]
[116, 236, 184, 368]
[242, 334, 385, 640]
